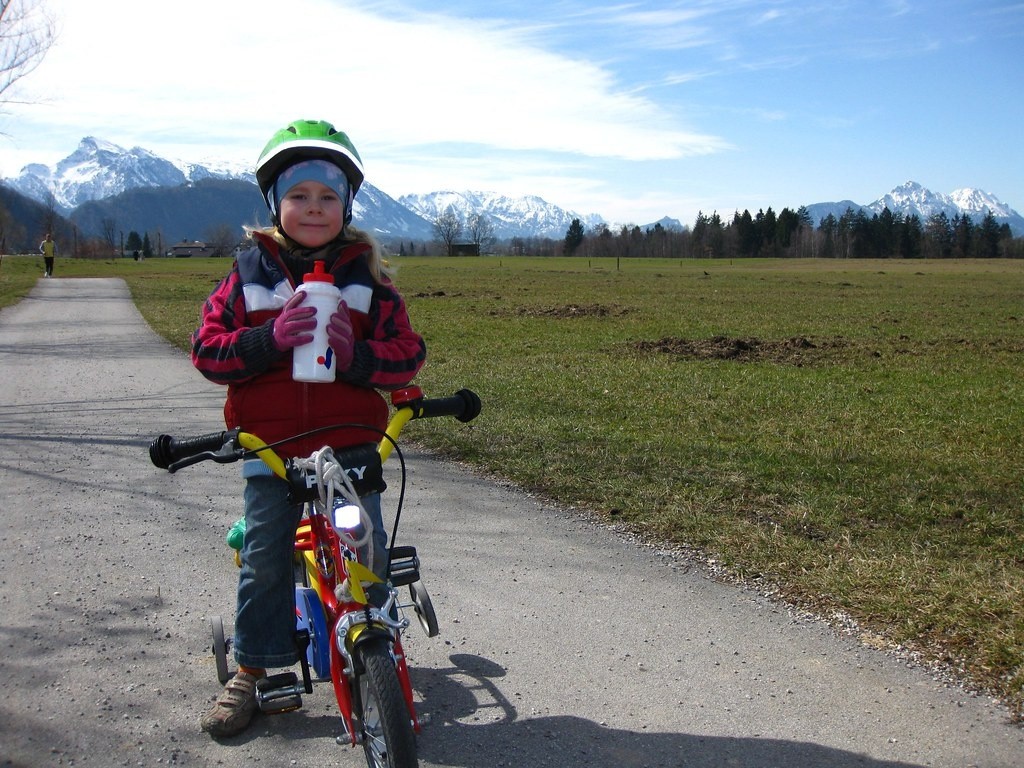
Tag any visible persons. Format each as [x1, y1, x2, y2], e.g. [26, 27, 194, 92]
[192, 120, 427, 731]
[39, 234, 58, 278]
[133, 249, 143, 262]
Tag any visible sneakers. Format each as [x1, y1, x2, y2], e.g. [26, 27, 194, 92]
[200, 671, 267, 737]
[45, 271, 52, 280]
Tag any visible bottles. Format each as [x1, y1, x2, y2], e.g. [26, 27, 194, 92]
[292, 260, 341, 383]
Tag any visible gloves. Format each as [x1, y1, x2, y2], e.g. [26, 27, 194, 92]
[272, 290, 318, 353]
[326, 299, 355, 373]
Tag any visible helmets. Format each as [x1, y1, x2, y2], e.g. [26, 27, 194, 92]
[254, 118, 366, 251]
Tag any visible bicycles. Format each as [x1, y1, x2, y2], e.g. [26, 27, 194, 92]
[147, 383, 482, 767]
